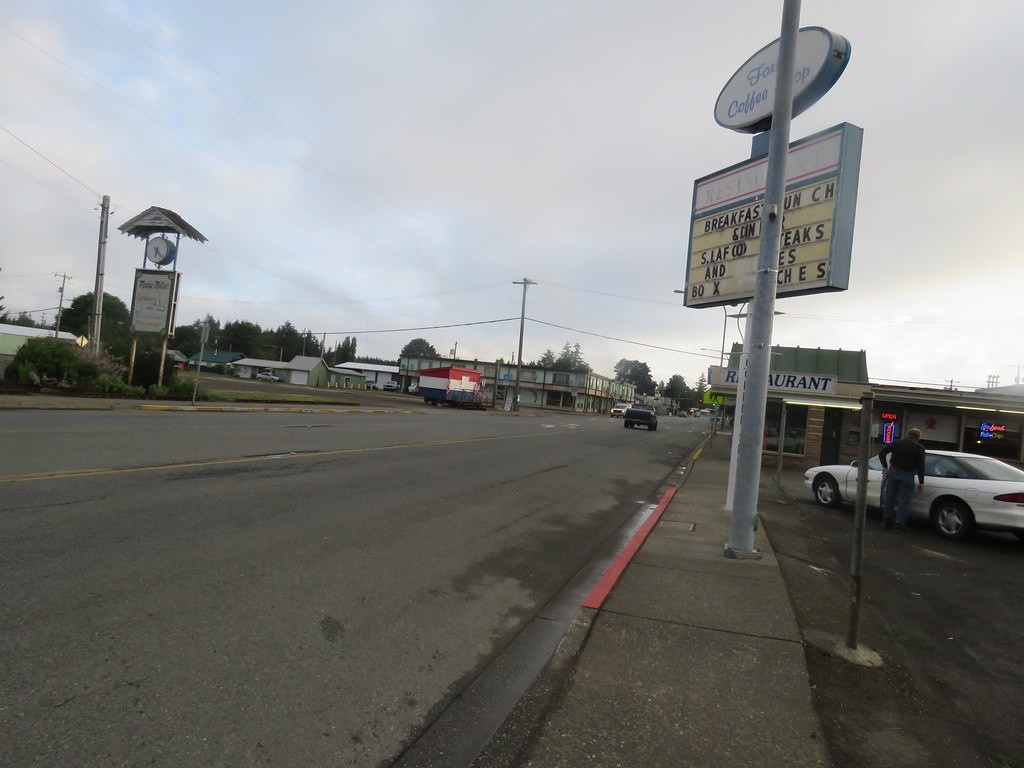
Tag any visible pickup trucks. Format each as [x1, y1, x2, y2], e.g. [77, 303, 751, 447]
[624, 404, 659, 430]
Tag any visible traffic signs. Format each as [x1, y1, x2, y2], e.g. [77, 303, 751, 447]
[128, 267, 177, 341]
[682, 122, 868, 308]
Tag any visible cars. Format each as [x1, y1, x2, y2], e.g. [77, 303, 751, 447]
[611, 402, 632, 418]
[365, 379, 377, 389]
[383, 380, 399, 390]
[256, 372, 278, 382]
[680, 408, 714, 417]
[803, 445, 1024, 539]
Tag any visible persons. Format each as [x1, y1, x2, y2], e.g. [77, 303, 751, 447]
[879, 428, 925, 532]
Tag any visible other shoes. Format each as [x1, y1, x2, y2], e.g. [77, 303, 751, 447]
[893, 523, 911, 533]
[881, 519, 891, 527]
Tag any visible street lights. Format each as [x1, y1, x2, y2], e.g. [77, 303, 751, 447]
[675, 288, 727, 368]
